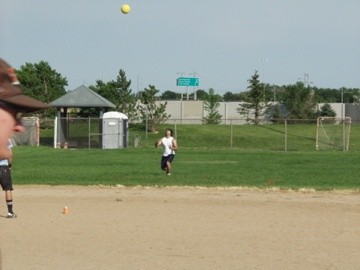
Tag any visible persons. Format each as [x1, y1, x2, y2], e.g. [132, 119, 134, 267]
[0, 58, 48, 218]
[155, 129, 178, 175]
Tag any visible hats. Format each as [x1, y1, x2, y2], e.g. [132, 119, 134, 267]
[0, 57, 50, 113]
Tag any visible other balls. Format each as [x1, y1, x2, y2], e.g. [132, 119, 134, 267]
[121, 5, 131, 14]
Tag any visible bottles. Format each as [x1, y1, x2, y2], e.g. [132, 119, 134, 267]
[64, 206, 68, 214]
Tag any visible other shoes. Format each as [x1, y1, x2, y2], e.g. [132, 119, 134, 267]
[167, 172, 172, 176]
[8, 212, 17, 218]
